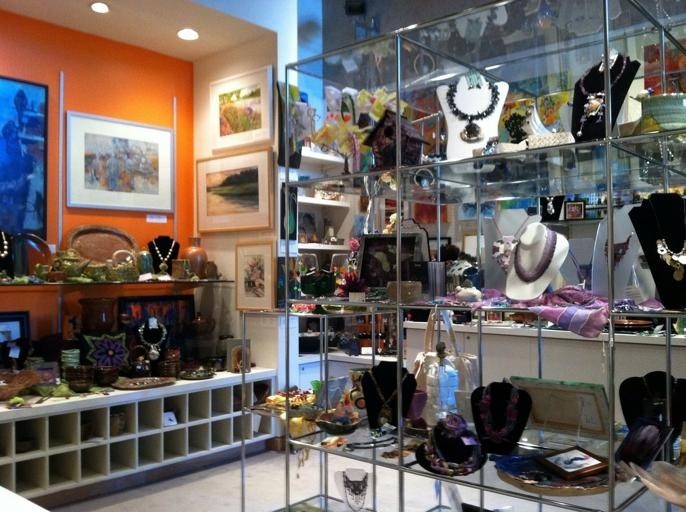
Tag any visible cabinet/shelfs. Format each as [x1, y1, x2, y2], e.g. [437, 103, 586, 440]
[0, 279, 234, 380]
[295, 193, 351, 250]
[1, 366, 276, 510]
[279, 1, 686, 512]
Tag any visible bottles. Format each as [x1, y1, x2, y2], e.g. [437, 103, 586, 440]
[181, 237, 208, 279]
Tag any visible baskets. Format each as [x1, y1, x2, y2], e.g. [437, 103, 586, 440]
[0, 372, 38, 401]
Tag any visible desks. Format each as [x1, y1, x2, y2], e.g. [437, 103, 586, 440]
[400, 320, 684, 428]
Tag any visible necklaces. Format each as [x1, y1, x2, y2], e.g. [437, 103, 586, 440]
[512, 232, 558, 285]
[489, 218, 531, 275]
[603, 230, 633, 274]
[575, 57, 628, 141]
[637, 375, 679, 429]
[647, 206, 685, 285]
[151, 237, 176, 273]
[447, 78, 503, 145]
[137, 318, 169, 363]
[342, 435, 404, 454]
[477, 383, 520, 445]
[367, 368, 410, 429]
[339, 470, 369, 512]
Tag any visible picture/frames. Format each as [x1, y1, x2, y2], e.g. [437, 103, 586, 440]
[191, 64, 276, 314]
[63, 108, 177, 217]
[0, 74, 49, 241]
